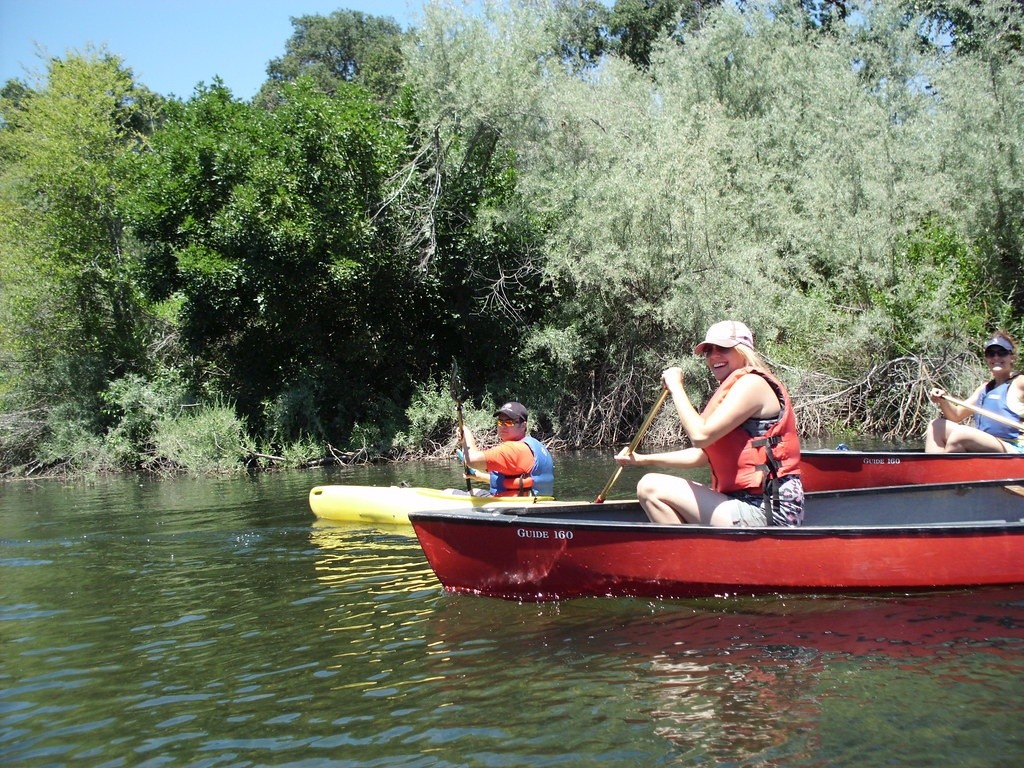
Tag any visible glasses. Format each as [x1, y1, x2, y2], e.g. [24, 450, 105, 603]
[497, 418, 519, 427]
[702, 343, 729, 358]
[985, 349, 1010, 358]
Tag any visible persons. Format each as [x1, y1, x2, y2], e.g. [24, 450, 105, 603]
[455, 402, 554, 498]
[614, 320, 805, 528]
[924, 328, 1024, 453]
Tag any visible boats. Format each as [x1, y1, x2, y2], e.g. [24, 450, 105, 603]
[796, 445, 1024, 486]
[309, 486, 637, 537]
[408, 483, 1024, 598]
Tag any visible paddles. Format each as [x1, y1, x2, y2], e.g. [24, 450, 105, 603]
[455, 403, 477, 495]
[936, 393, 1024, 434]
[589, 373, 676, 508]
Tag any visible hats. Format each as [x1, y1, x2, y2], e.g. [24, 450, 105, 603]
[493, 402, 528, 424]
[985, 336, 1013, 352]
[694, 320, 755, 354]
